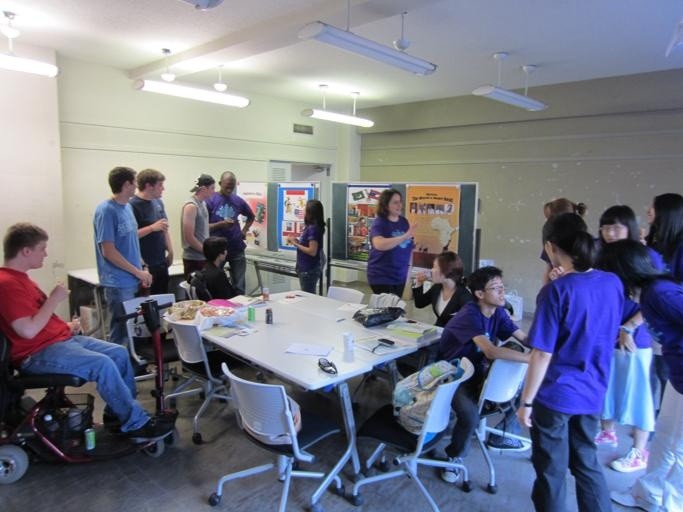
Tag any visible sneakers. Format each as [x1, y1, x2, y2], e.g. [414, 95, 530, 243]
[438, 453, 466, 485]
[606, 478, 666, 512]
[99, 412, 175, 444]
[486, 435, 533, 453]
[608, 446, 652, 476]
[593, 428, 619, 448]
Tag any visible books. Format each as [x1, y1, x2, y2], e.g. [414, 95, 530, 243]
[388, 322, 436, 342]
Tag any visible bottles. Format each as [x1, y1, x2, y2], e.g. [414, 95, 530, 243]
[43, 414, 59, 438]
[67, 407, 82, 432]
[265, 308, 273, 324]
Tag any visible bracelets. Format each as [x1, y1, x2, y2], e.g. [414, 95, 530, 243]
[142, 263, 151, 267]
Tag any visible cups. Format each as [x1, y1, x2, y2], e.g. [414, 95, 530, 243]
[417, 271, 431, 281]
[247, 306, 254, 321]
[262, 288, 269, 300]
[343, 334, 353, 353]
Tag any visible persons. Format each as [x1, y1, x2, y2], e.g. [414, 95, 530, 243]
[434, 265, 531, 484]
[181, 173, 215, 300]
[206, 170, 254, 295]
[0, 222, 175, 444]
[411, 251, 471, 371]
[128, 168, 175, 345]
[363, 189, 419, 298]
[285, 199, 326, 295]
[92, 165, 154, 348]
[203, 236, 240, 299]
[518, 192, 683, 512]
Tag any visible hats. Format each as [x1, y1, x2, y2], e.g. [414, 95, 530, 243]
[189, 173, 216, 193]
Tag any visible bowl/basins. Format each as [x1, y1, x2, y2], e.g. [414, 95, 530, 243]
[171, 299, 235, 327]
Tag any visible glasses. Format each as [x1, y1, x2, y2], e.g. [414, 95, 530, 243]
[599, 225, 626, 232]
[480, 284, 505, 292]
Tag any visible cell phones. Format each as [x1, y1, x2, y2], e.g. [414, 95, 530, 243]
[378, 339, 394, 346]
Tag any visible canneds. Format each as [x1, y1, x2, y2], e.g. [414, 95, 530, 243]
[140, 279, 147, 289]
[84, 428, 96, 450]
[416, 270, 432, 281]
[255, 202, 264, 219]
[68, 408, 83, 432]
[248, 306, 255, 322]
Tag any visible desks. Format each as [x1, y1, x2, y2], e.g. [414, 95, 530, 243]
[66, 265, 184, 322]
